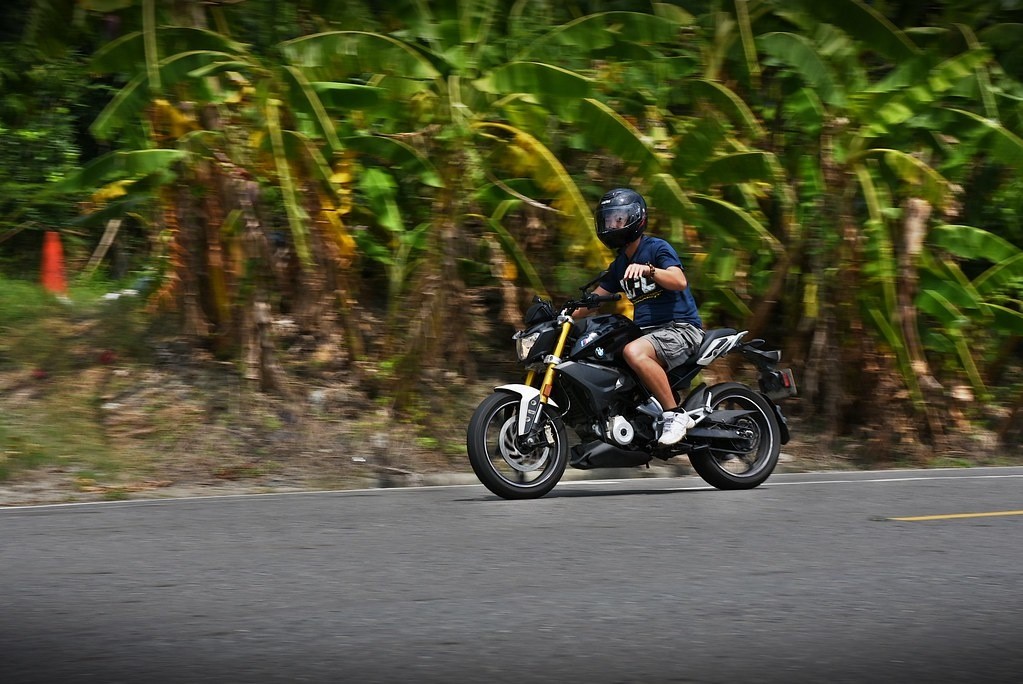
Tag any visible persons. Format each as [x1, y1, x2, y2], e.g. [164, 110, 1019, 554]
[524, 189, 704, 445]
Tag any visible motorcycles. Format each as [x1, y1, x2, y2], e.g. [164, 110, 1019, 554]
[463, 270, 799, 499]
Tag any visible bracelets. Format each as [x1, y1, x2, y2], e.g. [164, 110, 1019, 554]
[643, 262, 655, 279]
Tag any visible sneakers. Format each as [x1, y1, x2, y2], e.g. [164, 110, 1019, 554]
[657, 407, 696, 446]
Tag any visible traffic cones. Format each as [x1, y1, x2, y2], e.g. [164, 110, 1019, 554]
[39, 230, 66, 296]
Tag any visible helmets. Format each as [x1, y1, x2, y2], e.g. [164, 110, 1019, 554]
[595, 188, 647, 249]
